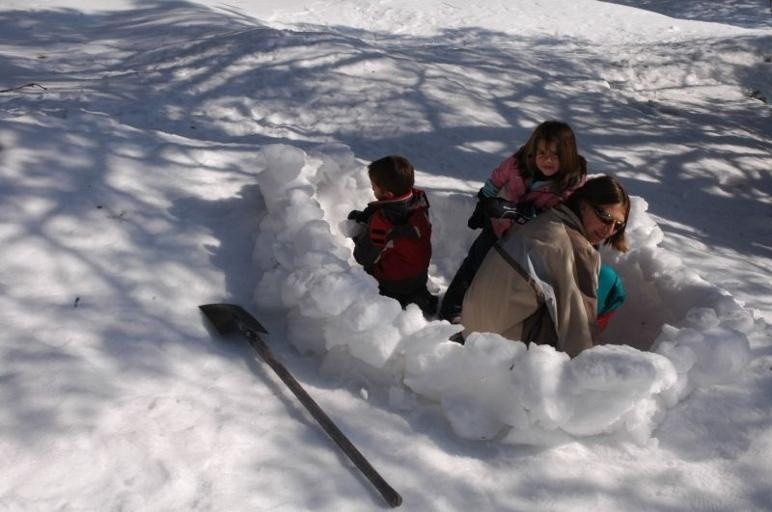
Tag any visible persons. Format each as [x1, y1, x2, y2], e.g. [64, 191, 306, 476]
[349, 155, 435, 318]
[456, 176, 632, 358]
[440, 118, 586, 324]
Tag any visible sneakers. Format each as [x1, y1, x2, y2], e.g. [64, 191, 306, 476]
[438, 310, 463, 325]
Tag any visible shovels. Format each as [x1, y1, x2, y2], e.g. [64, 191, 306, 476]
[198, 303, 403, 508]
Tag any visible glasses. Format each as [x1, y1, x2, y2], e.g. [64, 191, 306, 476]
[585, 199, 627, 233]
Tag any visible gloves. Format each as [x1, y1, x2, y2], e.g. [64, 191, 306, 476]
[345, 206, 370, 224]
[466, 187, 490, 230]
[484, 195, 535, 227]
